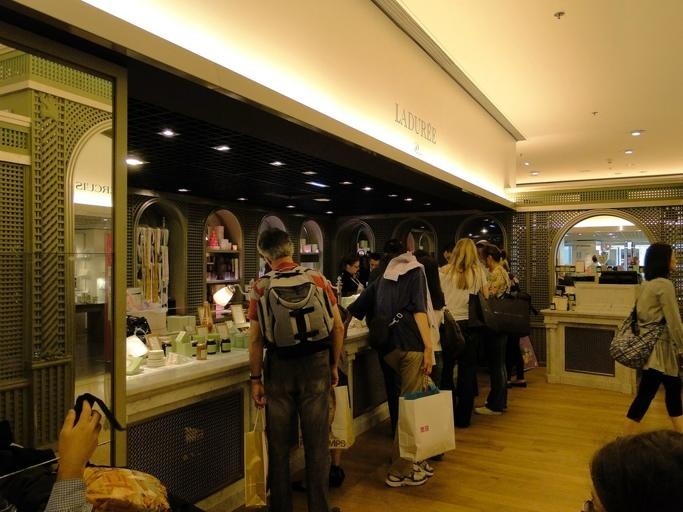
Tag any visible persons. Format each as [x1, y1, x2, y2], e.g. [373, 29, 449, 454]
[41, 400, 101, 512]
[582, 423, 683, 512]
[591, 255, 601, 267]
[607, 242, 682, 435]
[341, 236, 528, 487]
[248, 228, 343, 510]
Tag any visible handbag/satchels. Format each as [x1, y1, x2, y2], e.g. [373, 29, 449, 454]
[609, 311, 663, 370]
[490, 294, 532, 333]
[398, 390, 456, 461]
[244, 423, 271, 507]
[468, 292, 499, 334]
[440, 309, 465, 357]
[520, 335, 538, 371]
[84, 466, 170, 512]
[368, 315, 390, 350]
[298, 386, 356, 451]
[127, 314, 151, 336]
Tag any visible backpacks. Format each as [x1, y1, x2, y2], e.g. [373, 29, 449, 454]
[256, 266, 335, 350]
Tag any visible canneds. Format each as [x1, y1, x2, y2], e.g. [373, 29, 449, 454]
[196, 343, 207, 360]
[207, 338, 216, 354]
[162, 340, 173, 358]
[193, 341, 198, 356]
[221, 337, 231, 353]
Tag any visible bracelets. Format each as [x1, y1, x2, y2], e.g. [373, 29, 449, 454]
[250, 373, 262, 380]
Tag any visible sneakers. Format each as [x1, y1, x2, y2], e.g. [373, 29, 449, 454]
[385, 470, 427, 487]
[475, 406, 502, 416]
[411, 461, 435, 475]
[330, 467, 345, 486]
[512, 380, 527, 387]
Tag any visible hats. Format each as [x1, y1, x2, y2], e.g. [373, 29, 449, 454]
[384, 238, 405, 253]
[477, 239, 488, 247]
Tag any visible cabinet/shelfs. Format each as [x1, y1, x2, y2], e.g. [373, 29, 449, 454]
[204, 241, 242, 308]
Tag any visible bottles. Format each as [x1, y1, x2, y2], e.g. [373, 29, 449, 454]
[160, 337, 231, 360]
